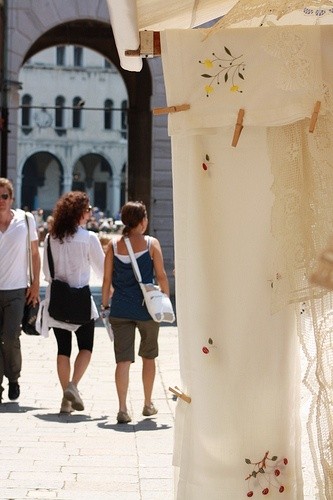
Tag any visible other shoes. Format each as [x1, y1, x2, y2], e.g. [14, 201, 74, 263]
[7, 381, 20, 400]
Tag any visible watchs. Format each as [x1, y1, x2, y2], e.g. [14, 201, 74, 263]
[101, 304, 110, 310]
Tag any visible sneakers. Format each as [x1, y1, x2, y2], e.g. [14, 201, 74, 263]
[64, 382, 84, 412]
[60, 399, 75, 414]
[142, 405, 159, 417]
[116, 411, 134, 423]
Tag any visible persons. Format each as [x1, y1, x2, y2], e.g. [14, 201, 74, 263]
[11, 205, 126, 248]
[0, 177, 42, 404]
[43, 190, 105, 414]
[101, 200, 170, 423]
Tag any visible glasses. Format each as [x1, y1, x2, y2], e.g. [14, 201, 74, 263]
[86, 206, 93, 212]
[1, 192, 9, 200]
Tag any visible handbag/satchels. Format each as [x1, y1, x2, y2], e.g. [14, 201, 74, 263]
[22, 299, 44, 335]
[48, 280, 91, 325]
[140, 282, 176, 324]
